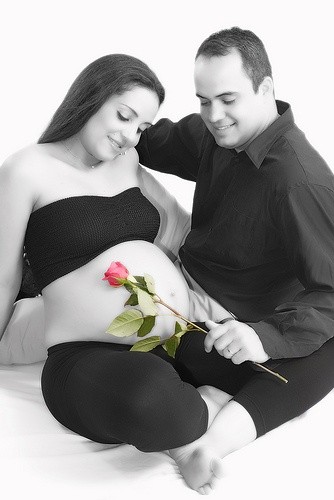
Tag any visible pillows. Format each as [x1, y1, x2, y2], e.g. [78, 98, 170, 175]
[0, 296, 47, 365]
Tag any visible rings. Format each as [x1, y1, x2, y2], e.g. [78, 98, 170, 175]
[226, 348, 234, 355]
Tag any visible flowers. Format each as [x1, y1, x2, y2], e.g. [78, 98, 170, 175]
[102, 260, 289, 384]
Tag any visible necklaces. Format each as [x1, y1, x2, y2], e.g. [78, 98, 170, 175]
[62, 139, 127, 168]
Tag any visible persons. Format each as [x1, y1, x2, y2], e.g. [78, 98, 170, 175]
[134, 26, 334, 364]
[0, 54, 334, 495]
